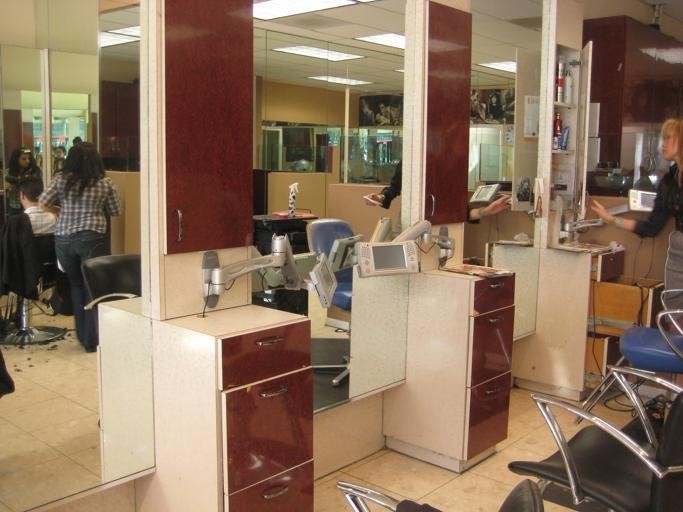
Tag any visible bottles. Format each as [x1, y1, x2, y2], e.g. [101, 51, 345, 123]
[556, 59, 574, 104]
[553, 113, 563, 150]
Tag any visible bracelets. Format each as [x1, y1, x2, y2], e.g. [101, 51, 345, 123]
[478, 207, 486, 218]
[612, 215, 624, 225]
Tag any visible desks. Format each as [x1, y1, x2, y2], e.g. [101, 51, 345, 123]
[254, 212, 319, 258]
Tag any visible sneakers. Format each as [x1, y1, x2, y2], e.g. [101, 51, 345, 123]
[83, 344, 98, 353]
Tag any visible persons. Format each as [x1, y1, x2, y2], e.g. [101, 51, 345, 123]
[359, 97, 403, 126]
[516, 177, 530, 202]
[470, 89, 505, 124]
[590, 118, 683, 421]
[364, 157, 401, 223]
[0, 136, 124, 352]
[534, 196, 543, 217]
[467, 194, 512, 222]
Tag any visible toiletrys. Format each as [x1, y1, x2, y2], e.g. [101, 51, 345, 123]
[561, 128, 572, 151]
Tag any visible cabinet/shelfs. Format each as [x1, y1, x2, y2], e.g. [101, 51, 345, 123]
[382, 266, 516, 473]
[483, 240, 537, 338]
[96, 297, 155, 485]
[550, 40, 592, 216]
[514, 241, 664, 402]
[157, 0, 254, 256]
[134, 305, 314, 512]
[416, 2, 472, 227]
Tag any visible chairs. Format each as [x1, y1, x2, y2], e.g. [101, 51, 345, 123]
[0, 214, 68, 345]
[81, 254, 141, 353]
[306, 217, 354, 387]
[296, 208, 311, 213]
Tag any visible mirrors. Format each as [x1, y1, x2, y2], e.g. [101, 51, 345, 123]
[0, 1, 148, 512]
[462, 0, 550, 342]
[252, 2, 415, 416]
